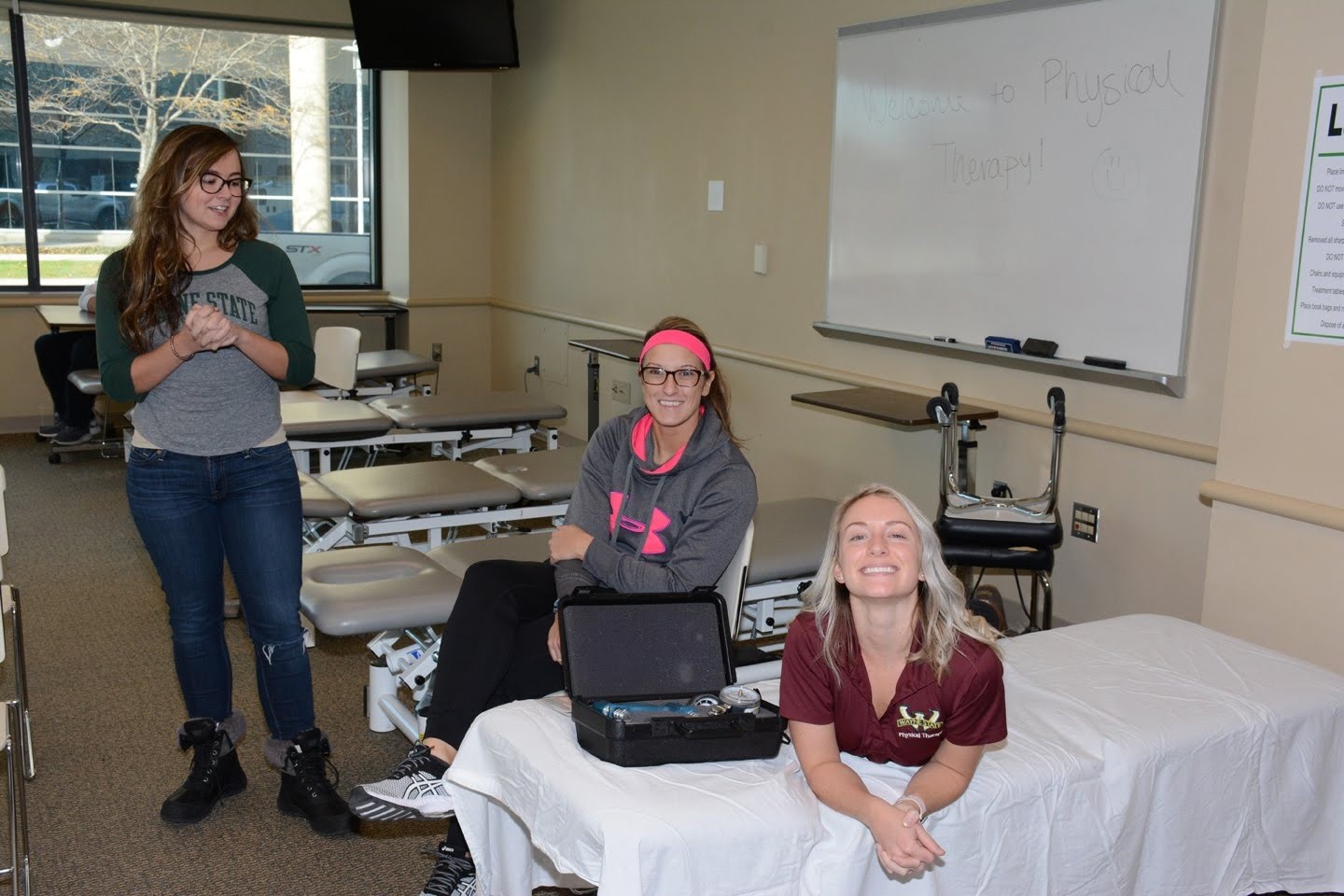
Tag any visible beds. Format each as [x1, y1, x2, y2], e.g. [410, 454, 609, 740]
[67, 347, 1344, 896]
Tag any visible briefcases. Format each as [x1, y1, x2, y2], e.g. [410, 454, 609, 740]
[558, 582, 793, 768]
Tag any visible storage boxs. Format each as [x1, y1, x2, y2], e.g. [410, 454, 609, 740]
[557, 585, 788, 767]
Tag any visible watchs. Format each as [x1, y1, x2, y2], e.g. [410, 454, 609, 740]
[553, 598, 560, 611]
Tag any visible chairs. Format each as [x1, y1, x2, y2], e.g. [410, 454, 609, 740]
[280, 327, 361, 403]
[714, 518, 754, 641]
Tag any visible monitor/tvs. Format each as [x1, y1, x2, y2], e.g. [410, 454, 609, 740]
[349, 0, 518, 70]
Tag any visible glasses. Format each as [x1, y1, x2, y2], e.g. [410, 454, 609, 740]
[640, 364, 704, 390]
[200, 172, 252, 199]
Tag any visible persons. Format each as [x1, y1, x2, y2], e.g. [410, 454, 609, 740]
[348, 314, 759, 896]
[96, 124, 358, 837]
[773, 484, 1010, 877]
[33, 283, 101, 446]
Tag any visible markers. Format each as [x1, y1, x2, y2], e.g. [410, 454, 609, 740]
[934, 338, 955, 342]
[1084, 358, 1126, 370]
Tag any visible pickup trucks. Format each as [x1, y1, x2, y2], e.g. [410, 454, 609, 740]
[0, 179, 126, 231]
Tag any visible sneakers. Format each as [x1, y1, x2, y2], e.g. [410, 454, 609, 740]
[349, 738, 456, 820]
[53, 425, 93, 445]
[419, 841, 478, 896]
[39, 418, 99, 439]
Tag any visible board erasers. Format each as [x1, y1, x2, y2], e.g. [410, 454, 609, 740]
[986, 336, 1021, 353]
[1022, 338, 1057, 357]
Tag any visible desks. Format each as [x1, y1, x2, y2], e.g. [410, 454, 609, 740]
[567, 339, 644, 442]
[35, 305, 96, 334]
[791, 386, 998, 600]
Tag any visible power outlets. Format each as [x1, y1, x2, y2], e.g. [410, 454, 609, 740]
[534, 356, 539, 376]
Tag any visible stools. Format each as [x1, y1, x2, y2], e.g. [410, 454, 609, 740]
[932, 521, 1054, 636]
[927, 382, 1065, 548]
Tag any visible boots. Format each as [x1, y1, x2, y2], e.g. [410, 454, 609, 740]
[265, 727, 356, 836]
[160, 711, 249, 823]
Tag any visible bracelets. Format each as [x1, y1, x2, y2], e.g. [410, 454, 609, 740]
[897, 795, 928, 825]
[170, 332, 195, 361]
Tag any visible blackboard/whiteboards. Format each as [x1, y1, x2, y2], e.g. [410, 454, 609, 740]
[812, 1, 1222, 399]
